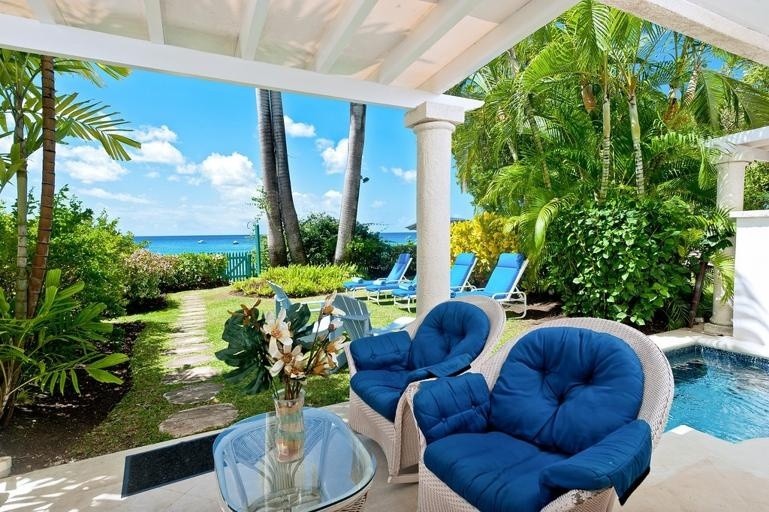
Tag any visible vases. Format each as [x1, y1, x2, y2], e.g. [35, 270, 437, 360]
[273, 389, 306, 464]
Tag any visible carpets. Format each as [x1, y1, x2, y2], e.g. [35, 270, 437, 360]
[119, 427, 250, 498]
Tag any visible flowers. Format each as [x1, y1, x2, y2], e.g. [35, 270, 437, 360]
[214, 289, 350, 409]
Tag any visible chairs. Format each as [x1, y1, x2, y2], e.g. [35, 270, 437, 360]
[341, 293, 507, 487]
[453, 254, 534, 320]
[393, 253, 478, 319]
[404, 314, 676, 512]
[365, 266, 416, 307]
[343, 252, 413, 303]
